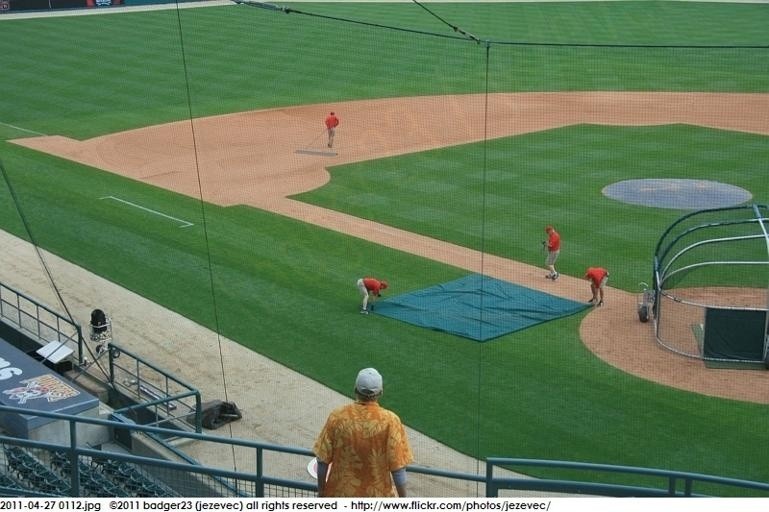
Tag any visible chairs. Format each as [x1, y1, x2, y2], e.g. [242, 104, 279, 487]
[0, 429, 178, 497]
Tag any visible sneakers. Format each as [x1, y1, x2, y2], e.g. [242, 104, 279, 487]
[546, 272, 559, 280]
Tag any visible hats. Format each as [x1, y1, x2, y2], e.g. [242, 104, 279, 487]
[355, 367, 384, 397]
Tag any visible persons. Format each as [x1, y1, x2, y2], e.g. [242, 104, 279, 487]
[325, 111, 339, 148]
[313, 368, 415, 497]
[357, 278, 388, 315]
[543, 226, 560, 280]
[583, 267, 609, 307]
